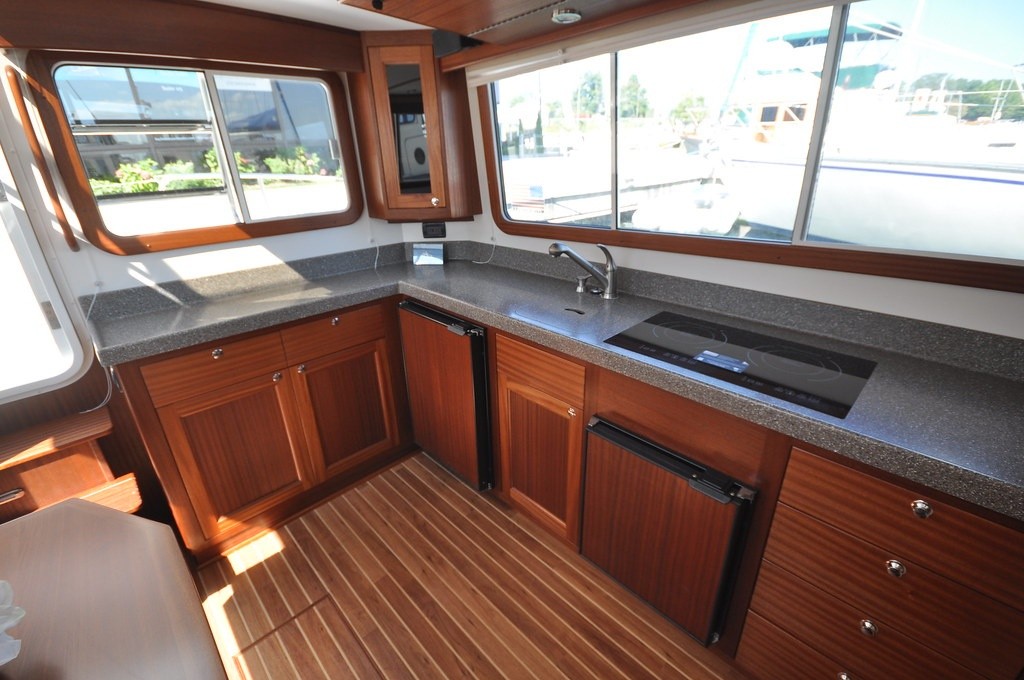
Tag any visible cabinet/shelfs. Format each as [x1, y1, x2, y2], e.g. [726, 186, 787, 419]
[368, 33, 468, 222]
[487, 328, 588, 556]
[402, 301, 494, 493]
[113, 298, 405, 569]
[577, 365, 771, 650]
[0, 400, 114, 520]
[711, 438, 1022, 680]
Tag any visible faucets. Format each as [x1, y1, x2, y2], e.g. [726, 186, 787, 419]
[548, 241, 618, 298]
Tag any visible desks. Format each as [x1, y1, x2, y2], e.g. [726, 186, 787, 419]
[2, 498, 230, 678]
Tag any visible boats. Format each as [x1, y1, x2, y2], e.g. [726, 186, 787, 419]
[654, 17, 1024, 260]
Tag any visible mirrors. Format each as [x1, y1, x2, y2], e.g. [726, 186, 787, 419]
[366, 46, 449, 211]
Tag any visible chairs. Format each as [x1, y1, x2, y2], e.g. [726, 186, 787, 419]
[27, 470, 142, 518]
